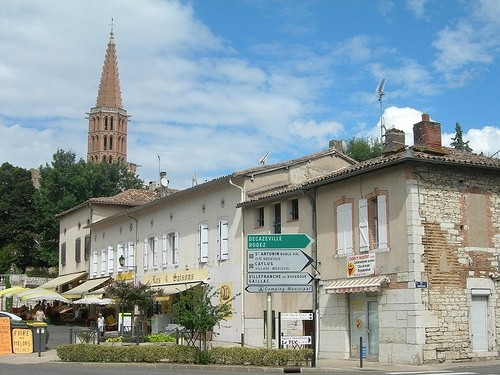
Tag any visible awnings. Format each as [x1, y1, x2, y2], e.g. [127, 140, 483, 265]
[62, 277, 133, 299]
[39, 272, 86, 289]
[325, 276, 385, 294]
[140, 281, 203, 296]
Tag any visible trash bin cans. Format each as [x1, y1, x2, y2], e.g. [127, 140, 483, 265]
[28, 322, 48, 353]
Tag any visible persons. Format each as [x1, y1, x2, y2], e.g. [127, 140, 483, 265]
[34, 299, 52, 316]
[97, 313, 105, 336]
[35, 306, 45, 321]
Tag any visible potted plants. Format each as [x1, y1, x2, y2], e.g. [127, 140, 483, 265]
[138, 331, 176, 347]
[100, 335, 137, 346]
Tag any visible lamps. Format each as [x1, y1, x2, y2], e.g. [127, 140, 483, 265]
[118, 255, 137, 274]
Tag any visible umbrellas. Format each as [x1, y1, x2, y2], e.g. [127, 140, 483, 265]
[0, 286, 70, 307]
[71, 297, 134, 318]
